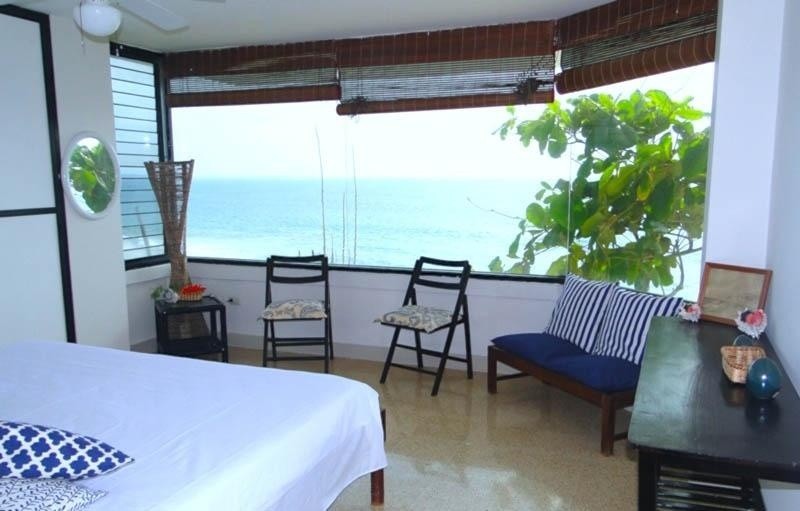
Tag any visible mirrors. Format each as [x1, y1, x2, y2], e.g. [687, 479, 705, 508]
[60, 130, 122, 220]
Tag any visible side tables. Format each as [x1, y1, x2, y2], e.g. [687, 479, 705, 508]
[154, 296, 229, 363]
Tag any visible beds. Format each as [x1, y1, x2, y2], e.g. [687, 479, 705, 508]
[1, 338, 386, 511]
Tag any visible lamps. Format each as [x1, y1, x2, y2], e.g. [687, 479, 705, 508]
[72, 0, 125, 58]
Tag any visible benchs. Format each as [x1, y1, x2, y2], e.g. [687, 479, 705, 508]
[485, 288, 694, 457]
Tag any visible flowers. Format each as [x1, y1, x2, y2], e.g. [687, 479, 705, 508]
[180, 283, 207, 294]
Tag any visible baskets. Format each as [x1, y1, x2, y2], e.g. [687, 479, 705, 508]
[721, 345, 766, 385]
[179, 291, 202, 301]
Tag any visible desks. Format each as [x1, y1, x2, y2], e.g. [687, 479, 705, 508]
[628, 314, 799, 511]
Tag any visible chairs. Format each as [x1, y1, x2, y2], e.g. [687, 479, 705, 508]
[374, 256, 473, 397]
[256, 253, 334, 373]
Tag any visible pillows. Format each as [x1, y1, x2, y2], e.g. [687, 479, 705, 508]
[544, 271, 686, 365]
[1, 419, 136, 510]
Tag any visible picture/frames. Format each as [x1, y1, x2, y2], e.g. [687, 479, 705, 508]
[699, 261, 773, 327]
